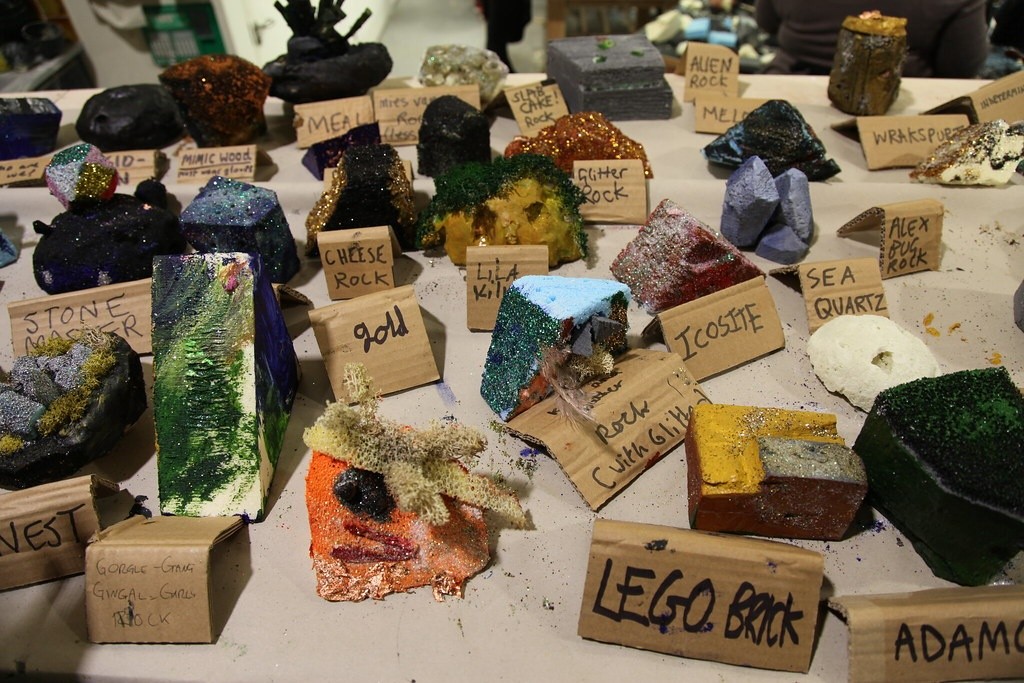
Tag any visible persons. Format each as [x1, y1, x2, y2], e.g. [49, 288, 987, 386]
[474, 1, 534, 73]
[755, 1, 990, 79]
